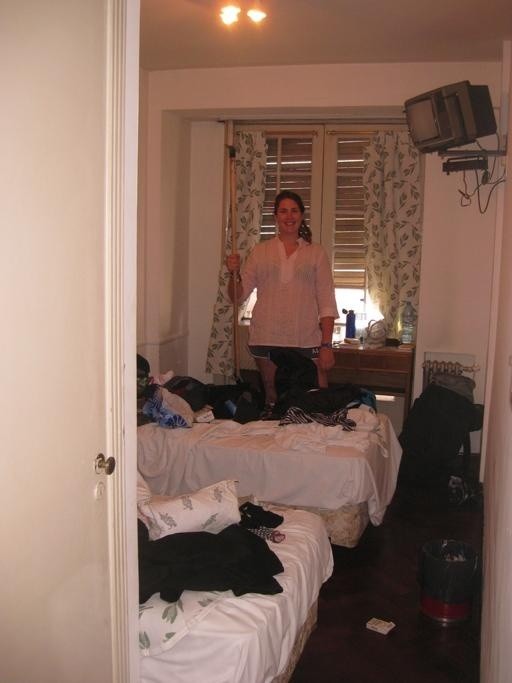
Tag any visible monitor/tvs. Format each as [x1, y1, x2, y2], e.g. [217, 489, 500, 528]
[405, 80, 498, 154]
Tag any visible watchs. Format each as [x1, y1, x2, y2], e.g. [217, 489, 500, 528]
[320, 340, 334, 350]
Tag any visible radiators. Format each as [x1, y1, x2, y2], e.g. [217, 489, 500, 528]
[422, 360, 463, 393]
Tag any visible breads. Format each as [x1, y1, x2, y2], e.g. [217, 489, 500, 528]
[344, 338, 360, 344]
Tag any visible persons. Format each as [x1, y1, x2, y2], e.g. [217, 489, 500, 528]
[225, 191, 339, 410]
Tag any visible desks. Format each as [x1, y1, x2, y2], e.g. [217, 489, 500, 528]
[315, 339, 414, 433]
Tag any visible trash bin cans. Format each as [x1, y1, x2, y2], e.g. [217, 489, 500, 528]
[415, 540, 479, 631]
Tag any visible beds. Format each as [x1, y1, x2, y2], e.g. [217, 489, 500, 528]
[137, 404, 389, 551]
[137, 494, 324, 682]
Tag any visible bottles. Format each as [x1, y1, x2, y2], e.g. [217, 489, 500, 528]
[400, 301, 416, 344]
[342, 308, 356, 337]
